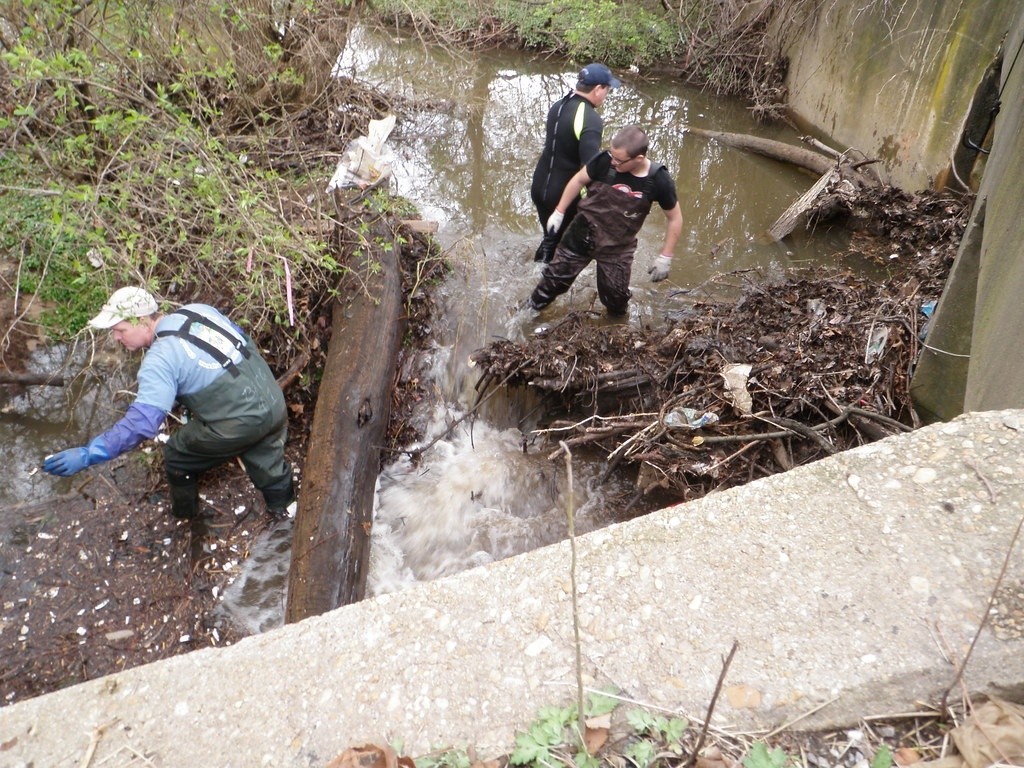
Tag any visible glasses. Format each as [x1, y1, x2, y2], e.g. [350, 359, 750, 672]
[607, 149, 634, 165]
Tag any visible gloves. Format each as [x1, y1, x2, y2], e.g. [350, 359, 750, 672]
[42, 402, 168, 476]
[547, 208, 564, 234]
[648, 255, 672, 282]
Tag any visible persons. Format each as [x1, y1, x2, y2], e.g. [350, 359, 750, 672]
[42, 286, 294, 517]
[529, 126, 682, 318]
[529, 63, 622, 264]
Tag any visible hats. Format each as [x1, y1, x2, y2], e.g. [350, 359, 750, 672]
[578, 63, 621, 88]
[91, 286, 159, 329]
